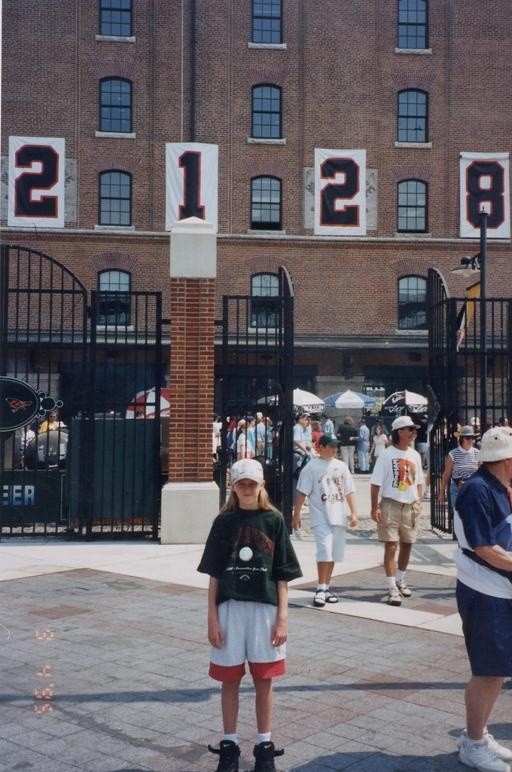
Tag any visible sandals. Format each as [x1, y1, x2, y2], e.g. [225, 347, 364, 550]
[324, 588, 339, 604]
[313, 589, 325, 606]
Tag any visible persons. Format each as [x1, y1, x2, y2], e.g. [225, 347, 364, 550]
[40, 411, 64, 435]
[214, 413, 509, 500]
[291, 432, 357, 607]
[370, 417, 424, 606]
[454, 427, 512, 771]
[196, 459, 302, 771]
[437, 424, 482, 509]
[20, 425, 34, 453]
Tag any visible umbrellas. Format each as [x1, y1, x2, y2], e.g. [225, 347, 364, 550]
[128, 387, 171, 418]
[323, 389, 377, 413]
[271, 388, 325, 412]
[382, 389, 428, 416]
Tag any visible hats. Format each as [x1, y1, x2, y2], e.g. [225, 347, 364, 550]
[478, 426, 512, 462]
[229, 459, 264, 485]
[391, 416, 421, 432]
[320, 435, 343, 447]
[454, 425, 481, 439]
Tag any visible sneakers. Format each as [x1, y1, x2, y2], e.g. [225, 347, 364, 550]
[252, 742, 285, 772]
[208, 740, 242, 772]
[462, 727, 512, 761]
[388, 587, 402, 606]
[396, 579, 412, 597]
[458, 736, 511, 772]
